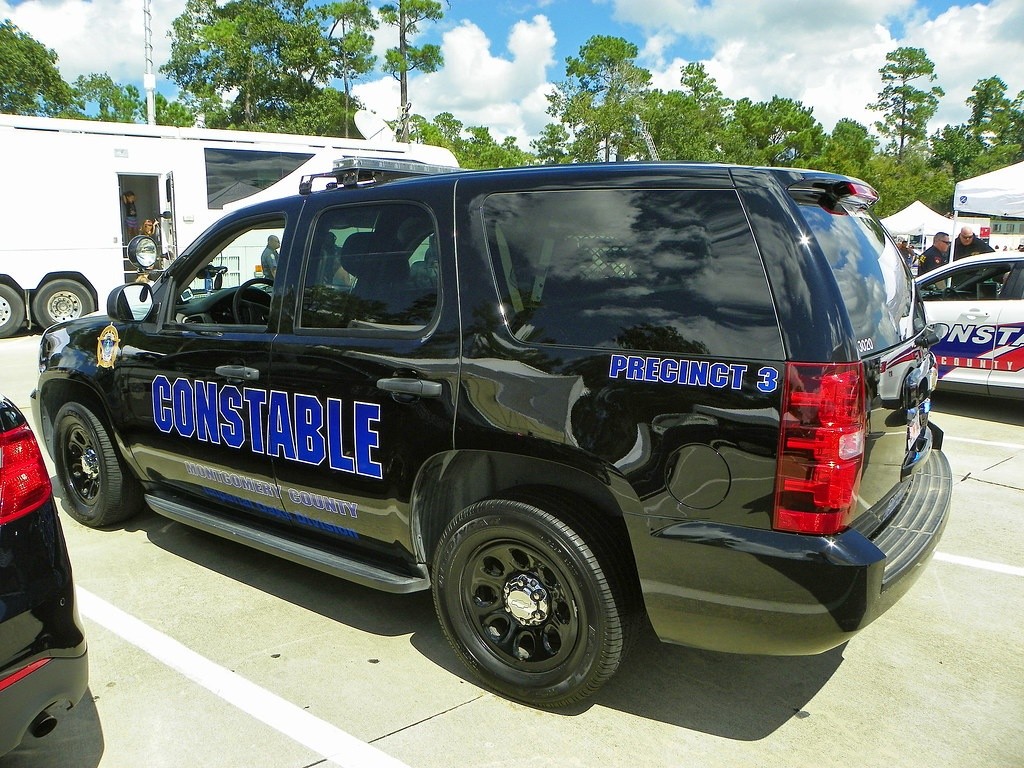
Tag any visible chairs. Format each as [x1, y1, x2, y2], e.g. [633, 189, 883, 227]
[341, 233, 413, 328]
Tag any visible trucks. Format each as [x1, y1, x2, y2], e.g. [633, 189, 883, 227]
[0, 110, 464, 337]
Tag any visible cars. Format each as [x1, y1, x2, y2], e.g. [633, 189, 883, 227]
[0, 392, 108, 767]
[914, 251, 1024, 403]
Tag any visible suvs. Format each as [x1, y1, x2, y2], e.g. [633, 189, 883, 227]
[22, 160, 957, 712]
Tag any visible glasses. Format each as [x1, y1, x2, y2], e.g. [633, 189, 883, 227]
[940, 240, 951, 246]
[961, 234, 973, 239]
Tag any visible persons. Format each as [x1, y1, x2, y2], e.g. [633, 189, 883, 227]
[261, 235, 280, 281]
[899, 241, 916, 266]
[121, 191, 138, 242]
[140, 219, 159, 236]
[918, 232, 949, 290]
[946, 227, 996, 291]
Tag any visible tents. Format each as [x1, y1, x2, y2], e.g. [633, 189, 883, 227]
[878, 200, 980, 250]
[223, 144, 345, 280]
[946, 161, 1024, 289]
